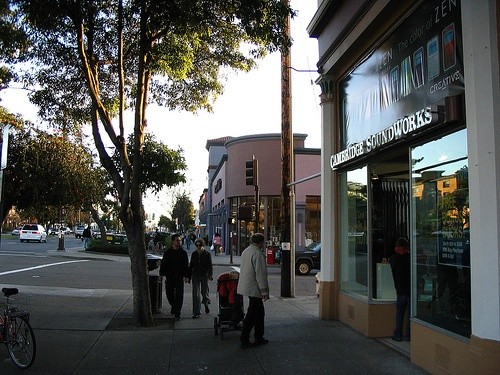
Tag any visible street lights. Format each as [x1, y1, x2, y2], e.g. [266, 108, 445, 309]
[86, 146, 115, 225]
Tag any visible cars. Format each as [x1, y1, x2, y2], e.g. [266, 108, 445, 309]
[20, 223, 48, 242]
[47, 227, 70, 237]
[11, 226, 23, 236]
[294, 241, 322, 276]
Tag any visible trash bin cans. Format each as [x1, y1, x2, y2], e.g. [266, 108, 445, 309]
[146, 254, 164, 314]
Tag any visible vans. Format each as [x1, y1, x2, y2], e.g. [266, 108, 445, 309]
[75, 226, 85, 239]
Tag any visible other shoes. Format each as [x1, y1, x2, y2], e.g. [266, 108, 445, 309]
[205, 305, 210, 314]
[193, 313, 200, 319]
[170, 308, 181, 319]
[240, 341, 254, 349]
[255, 338, 269, 346]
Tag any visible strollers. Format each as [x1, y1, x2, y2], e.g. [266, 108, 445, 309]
[212, 271, 246, 340]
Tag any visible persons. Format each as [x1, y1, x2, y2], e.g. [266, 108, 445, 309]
[416, 244, 427, 293]
[188, 239, 213, 319]
[238, 233, 270, 349]
[462, 231, 470, 290]
[214, 233, 221, 256]
[178, 233, 196, 251]
[432, 233, 460, 307]
[83, 226, 92, 250]
[221, 271, 241, 326]
[162, 234, 188, 320]
[391, 236, 411, 342]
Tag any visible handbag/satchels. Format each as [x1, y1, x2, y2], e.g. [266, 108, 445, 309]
[219, 246, 224, 254]
[210, 244, 215, 251]
[213, 239, 217, 244]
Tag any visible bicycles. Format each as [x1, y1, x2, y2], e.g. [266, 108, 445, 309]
[0, 287, 36, 369]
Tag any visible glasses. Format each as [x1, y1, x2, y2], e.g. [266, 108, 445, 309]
[196, 245, 203, 247]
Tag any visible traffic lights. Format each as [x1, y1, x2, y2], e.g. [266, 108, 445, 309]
[245, 154, 255, 185]
